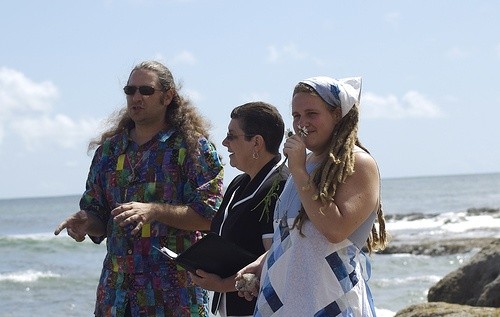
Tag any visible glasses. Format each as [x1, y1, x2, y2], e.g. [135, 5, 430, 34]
[227, 132, 254, 141]
[123, 85, 161, 95]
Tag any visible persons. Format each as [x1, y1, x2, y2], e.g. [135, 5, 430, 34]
[235, 76, 387, 317]
[187, 101, 293, 317]
[54, 60, 225, 317]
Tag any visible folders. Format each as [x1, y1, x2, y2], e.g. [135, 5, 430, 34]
[152, 231, 259, 279]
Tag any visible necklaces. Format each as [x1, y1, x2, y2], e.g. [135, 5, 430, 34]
[121, 129, 164, 185]
